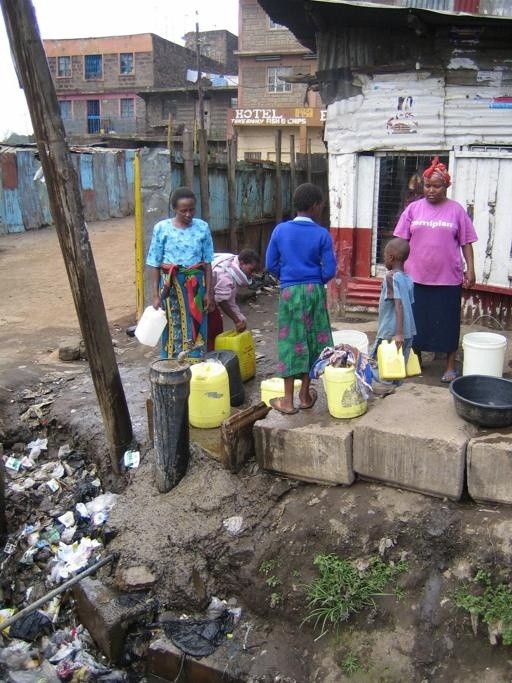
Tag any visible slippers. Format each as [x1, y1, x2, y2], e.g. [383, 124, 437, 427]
[441, 368, 460, 383]
[271, 397, 299, 415]
[300, 387, 318, 409]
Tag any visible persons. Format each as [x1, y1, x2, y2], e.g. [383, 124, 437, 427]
[367, 238, 418, 387]
[264, 184, 338, 415]
[144, 187, 215, 358]
[392, 156, 478, 382]
[205, 248, 260, 353]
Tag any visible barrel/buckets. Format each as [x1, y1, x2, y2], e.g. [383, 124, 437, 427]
[215, 328, 257, 380]
[204, 349, 245, 406]
[407, 348, 421, 377]
[332, 329, 369, 355]
[377, 338, 406, 380]
[320, 363, 368, 420]
[461, 314, 507, 377]
[187, 361, 231, 429]
[260, 377, 302, 410]
[133, 306, 168, 347]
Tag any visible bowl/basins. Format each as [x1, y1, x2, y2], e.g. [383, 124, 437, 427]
[449, 374, 512, 429]
